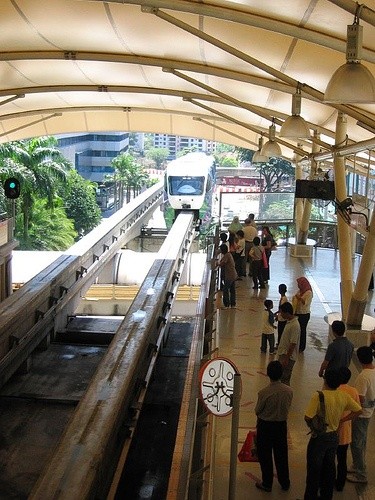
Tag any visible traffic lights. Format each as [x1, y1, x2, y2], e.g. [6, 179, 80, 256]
[4, 178, 21, 199]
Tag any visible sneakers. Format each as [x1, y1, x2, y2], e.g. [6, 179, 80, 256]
[347, 475, 368, 483]
[347, 465, 364, 473]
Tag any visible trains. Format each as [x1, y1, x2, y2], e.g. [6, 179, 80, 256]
[164, 150, 217, 210]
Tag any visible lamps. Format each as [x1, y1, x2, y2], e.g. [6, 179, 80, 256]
[260, 126, 282, 157]
[279, 94, 311, 138]
[322, 25, 375, 103]
[252, 138, 269, 163]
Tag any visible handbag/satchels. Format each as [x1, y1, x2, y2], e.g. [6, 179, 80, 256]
[272, 240, 277, 247]
[238, 431, 259, 462]
[234, 253, 243, 261]
[307, 391, 326, 435]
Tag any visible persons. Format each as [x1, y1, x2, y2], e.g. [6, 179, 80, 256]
[260, 300, 277, 356]
[256, 360, 293, 492]
[274, 284, 288, 349]
[277, 302, 300, 386]
[214, 213, 273, 310]
[296, 319, 375, 500]
[292, 276, 313, 353]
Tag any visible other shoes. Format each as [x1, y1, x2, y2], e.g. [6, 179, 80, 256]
[261, 347, 265, 352]
[255, 482, 272, 492]
[230, 305, 235, 308]
[281, 480, 290, 491]
[264, 281, 268, 283]
[259, 286, 265, 288]
[270, 350, 276, 355]
[221, 306, 228, 309]
[253, 287, 258, 289]
